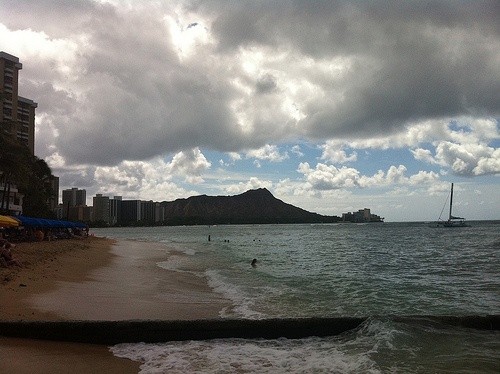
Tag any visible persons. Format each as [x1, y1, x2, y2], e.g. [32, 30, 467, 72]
[208, 235, 210, 241]
[250, 259, 257, 266]
[0, 239, 24, 268]
[85, 224, 89, 236]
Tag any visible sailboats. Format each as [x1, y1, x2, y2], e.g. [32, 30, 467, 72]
[423, 183, 472, 228]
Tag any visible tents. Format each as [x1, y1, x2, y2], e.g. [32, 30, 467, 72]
[17, 215, 86, 241]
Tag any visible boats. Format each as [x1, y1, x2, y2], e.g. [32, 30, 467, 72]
[336, 208, 385, 223]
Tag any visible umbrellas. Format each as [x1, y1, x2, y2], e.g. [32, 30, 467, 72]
[0, 214, 22, 239]
